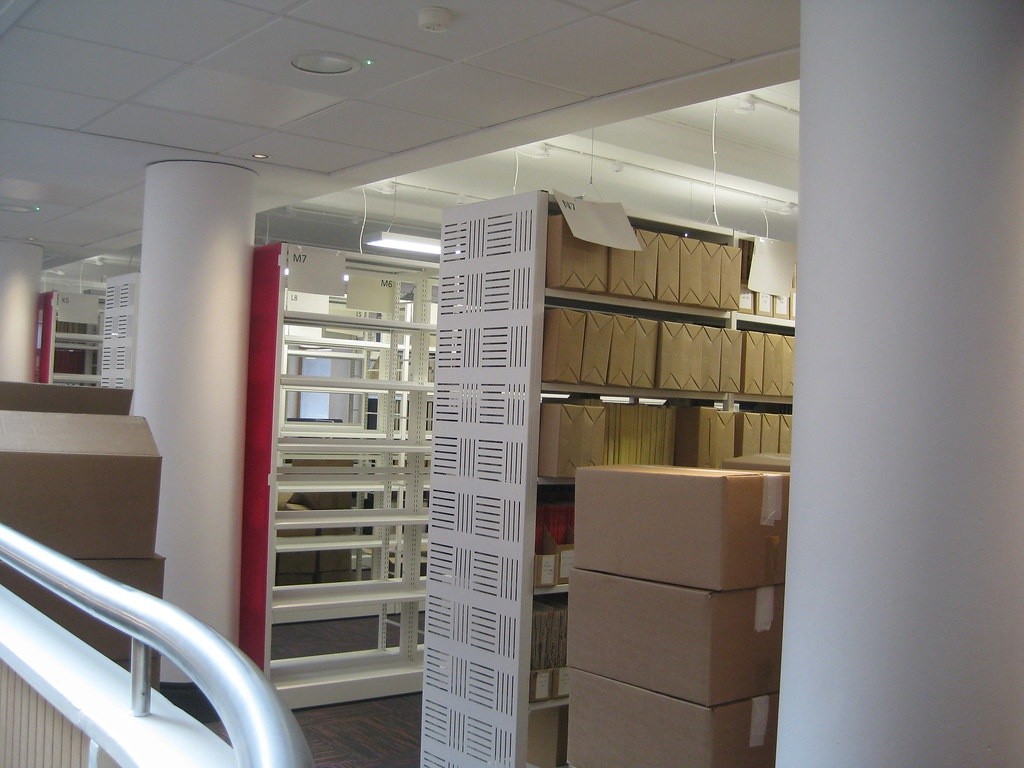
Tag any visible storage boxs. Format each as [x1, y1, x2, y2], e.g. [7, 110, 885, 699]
[0, 380, 165, 560]
[533, 190, 805, 768]
[0, 551, 167, 662]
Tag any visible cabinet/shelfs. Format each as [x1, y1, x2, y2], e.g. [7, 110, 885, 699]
[35, 289, 104, 387]
[240, 243, 443, 714]
